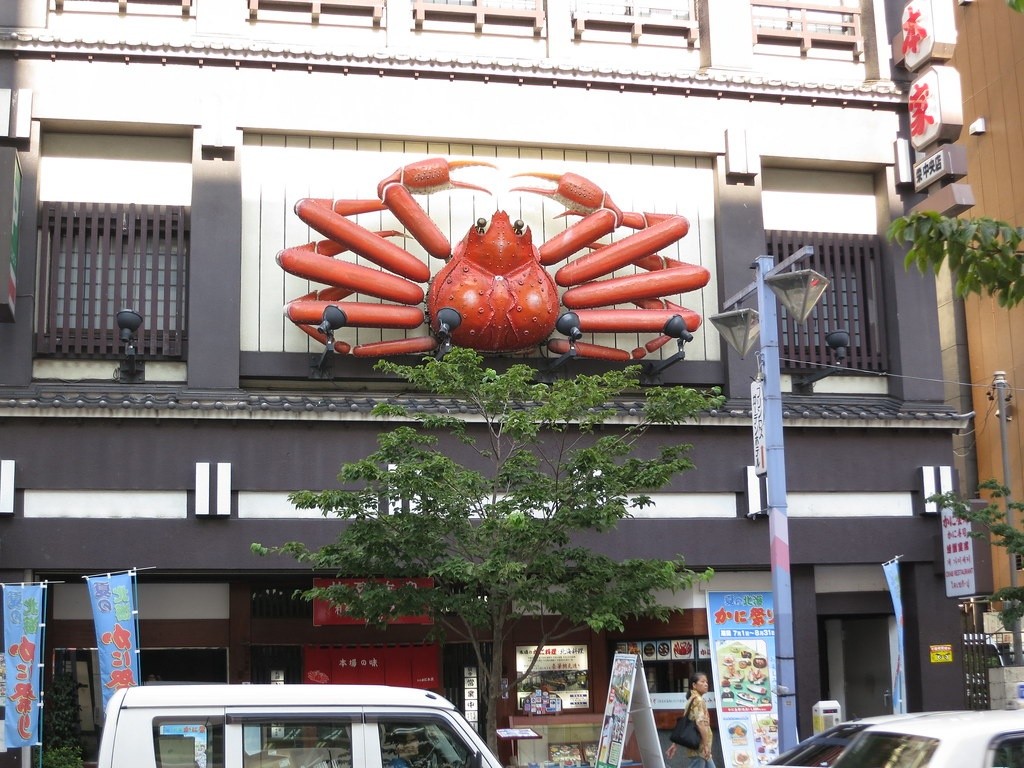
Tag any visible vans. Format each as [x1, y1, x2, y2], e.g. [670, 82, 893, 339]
[99, 684, 505, 768]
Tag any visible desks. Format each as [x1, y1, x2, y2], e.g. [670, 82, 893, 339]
[496, 727, 542, 756]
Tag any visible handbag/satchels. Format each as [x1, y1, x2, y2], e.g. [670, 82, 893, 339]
[670, 697, 702, 751]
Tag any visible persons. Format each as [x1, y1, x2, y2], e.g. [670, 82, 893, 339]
[665, 672, 717, 768]
[388, 729, 421, 768]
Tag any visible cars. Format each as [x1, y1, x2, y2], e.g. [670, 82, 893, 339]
[763, 709, 1024, 768]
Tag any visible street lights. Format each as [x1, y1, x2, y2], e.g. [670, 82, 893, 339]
[708, 245, 831, 757]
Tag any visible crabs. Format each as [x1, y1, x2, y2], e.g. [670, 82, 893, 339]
[274, 157, 711, 361]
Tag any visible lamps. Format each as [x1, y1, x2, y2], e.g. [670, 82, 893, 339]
[113, 311, 147, 383]
[540, 310, 580, 374]
[645, 312, 697, 377]
[428, 306, 462, 360]
[317, 305, 348, 368]
[800, 329, 852, 389]
